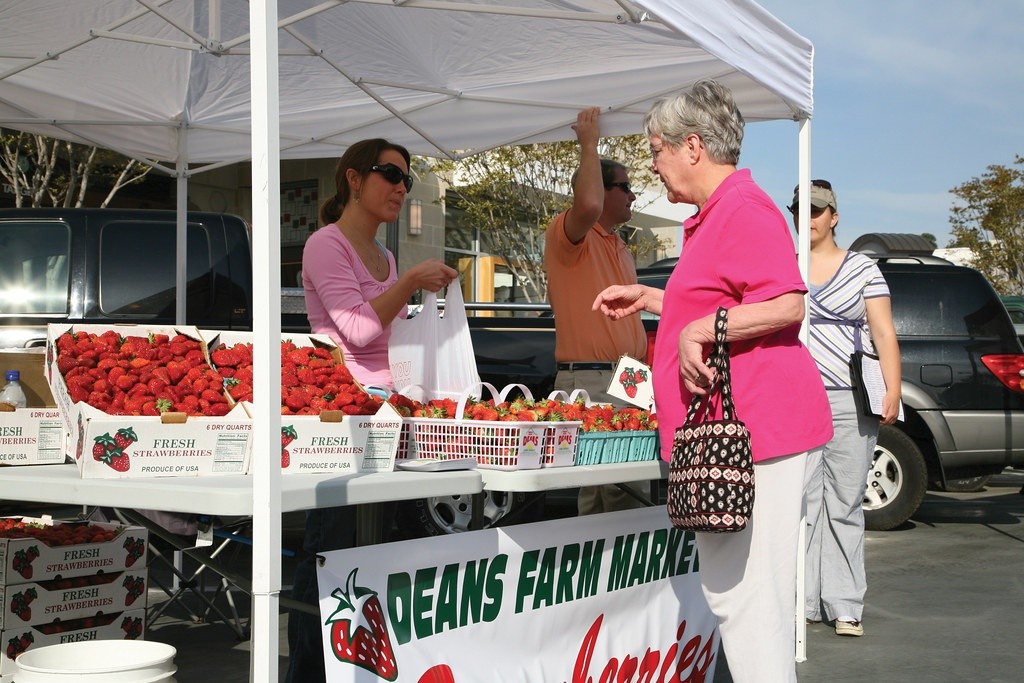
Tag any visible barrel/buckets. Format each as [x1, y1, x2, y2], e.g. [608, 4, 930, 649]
[13, 638, 178, 683]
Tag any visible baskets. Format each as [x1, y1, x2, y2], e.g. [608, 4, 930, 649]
[395, 382, 612, 472]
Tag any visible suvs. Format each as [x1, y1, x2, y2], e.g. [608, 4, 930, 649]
[538, 264, 1024, 532]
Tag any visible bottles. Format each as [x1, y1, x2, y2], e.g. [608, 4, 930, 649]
[0, 370, 27, 408]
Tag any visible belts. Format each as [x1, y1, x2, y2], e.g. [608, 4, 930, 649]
[557, 363, 616, 371]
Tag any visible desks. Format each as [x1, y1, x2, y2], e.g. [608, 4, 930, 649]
[0, 462, 483, 683]
[466, 459, 679, 528]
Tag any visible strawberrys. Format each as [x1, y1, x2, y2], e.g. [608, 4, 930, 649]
[0, 519, 117, 634]
[56, 330, 253, 416]
[281, 339, 380, 415]
[369, 392, 658, 465]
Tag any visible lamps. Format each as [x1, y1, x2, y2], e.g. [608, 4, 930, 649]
[407, 198, 422, 235]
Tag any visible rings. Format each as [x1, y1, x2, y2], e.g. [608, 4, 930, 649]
[695, 373, 701, 381]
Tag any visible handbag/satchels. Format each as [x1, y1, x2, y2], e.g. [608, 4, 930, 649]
[388, 277, 483, 404]
[667, 305, 755, 534]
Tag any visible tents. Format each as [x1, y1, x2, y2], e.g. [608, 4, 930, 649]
[0, 0, 814, 683]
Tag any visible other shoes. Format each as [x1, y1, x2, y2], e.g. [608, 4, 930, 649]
[836, 620, 864, 637]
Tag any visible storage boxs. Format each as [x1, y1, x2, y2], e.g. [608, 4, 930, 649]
[0, 322, 402, 479]
[0, 515, 149, 683]
[602, 354, 660, 420]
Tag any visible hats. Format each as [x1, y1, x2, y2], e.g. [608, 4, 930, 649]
[790, 186, 837, 210]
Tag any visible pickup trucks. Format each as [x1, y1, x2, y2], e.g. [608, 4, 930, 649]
[0, 207, 558, 535]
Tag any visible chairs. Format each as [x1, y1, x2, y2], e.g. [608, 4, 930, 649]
[84, 504, 252, 641]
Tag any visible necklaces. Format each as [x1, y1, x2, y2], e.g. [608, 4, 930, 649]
[338, 221, 382, 273]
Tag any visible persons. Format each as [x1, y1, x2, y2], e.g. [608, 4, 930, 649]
[545, 109, 650, 518]
[786, 180, 902, 636]
[286, 138, 461, 683]
[591, 82, 834, 683]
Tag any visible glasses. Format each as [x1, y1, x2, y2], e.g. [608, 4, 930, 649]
[651, 134, 681, 159]
[604, 182, 631, 193]
[357, 163, 413, 194]
